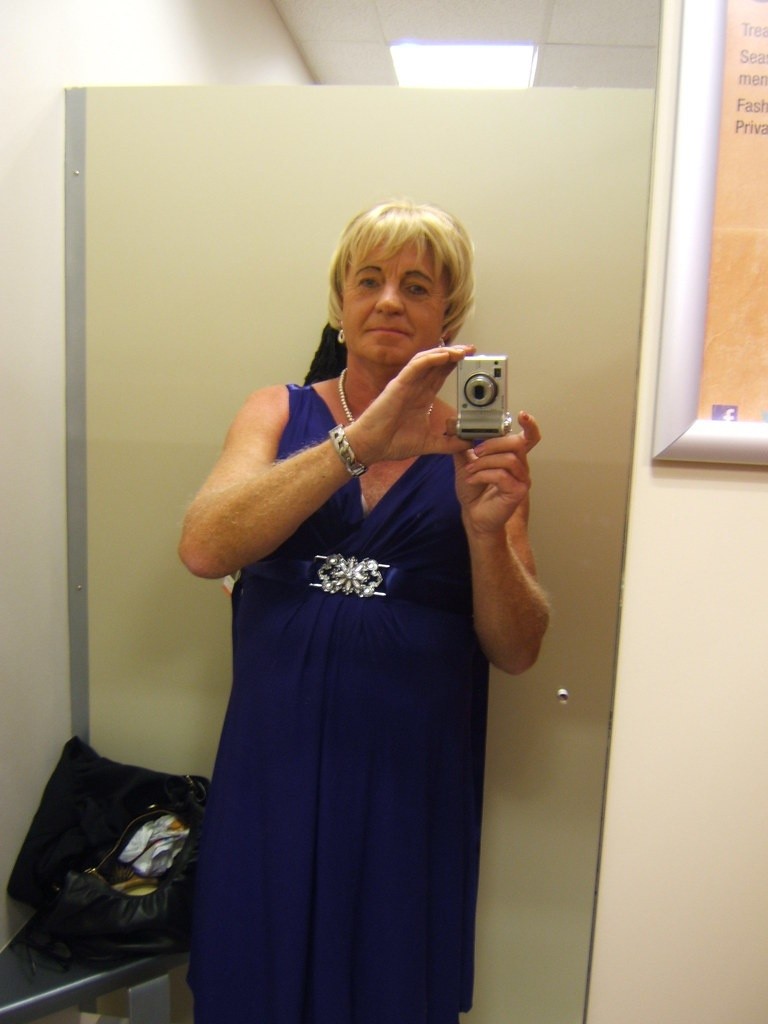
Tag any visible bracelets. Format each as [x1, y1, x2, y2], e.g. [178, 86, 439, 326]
[328, 422, 367, 478]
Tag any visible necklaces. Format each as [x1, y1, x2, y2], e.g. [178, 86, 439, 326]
[339, 364, 433, 426]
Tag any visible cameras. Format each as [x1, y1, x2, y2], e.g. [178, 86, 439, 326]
[454, 356, 511, 438]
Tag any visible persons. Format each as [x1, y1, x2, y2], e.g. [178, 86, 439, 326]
[180, 200, 541, 1023]
